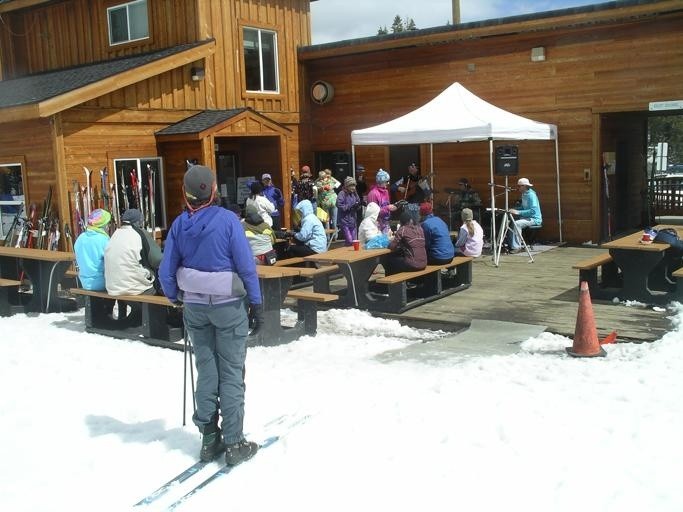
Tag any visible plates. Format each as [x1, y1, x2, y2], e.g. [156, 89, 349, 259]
[641, 241, 653, 244]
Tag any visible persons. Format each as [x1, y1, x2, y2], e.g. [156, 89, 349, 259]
[104, 209, 162, 297]
[392, 162, 431, 221]
[75, 208, 110, 292]
[452, 208, 484, 258]
[508, 177, 543, 254]
[419, 203, 454, 264]
[389, 212, 428, 272]
[227, 164, 398, 267]
[454, 178, 481, 215]
[158, 164, 264, 465]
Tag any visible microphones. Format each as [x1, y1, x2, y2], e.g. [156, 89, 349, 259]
[408, 174, 412, 178]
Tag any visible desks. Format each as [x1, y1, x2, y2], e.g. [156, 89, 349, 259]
[304, 243, 395, 308]
[0, 244, 78, 313]
[601, 224, 682, 310]
[246, 263, 316, 347]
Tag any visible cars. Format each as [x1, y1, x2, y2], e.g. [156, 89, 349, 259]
[646, 173, 682, 206]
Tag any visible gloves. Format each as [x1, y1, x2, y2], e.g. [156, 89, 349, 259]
[285, 231, 296, 237]
[352, 201, 360, 208]
[248, 302, 266, 337]
[360, 201, 368, 207]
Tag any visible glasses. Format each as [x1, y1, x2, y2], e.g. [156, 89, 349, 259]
[385, 181, 390, 183]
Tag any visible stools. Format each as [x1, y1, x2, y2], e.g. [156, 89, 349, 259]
[521, 225, 543, 251]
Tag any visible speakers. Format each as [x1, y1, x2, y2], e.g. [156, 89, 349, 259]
[496, 146, 518, 177]
[330, 152, 356, 191]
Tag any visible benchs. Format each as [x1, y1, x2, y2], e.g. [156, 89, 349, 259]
[69, 287, 194, 354]
[58, 270, 87, 307]
[0, 278, 21, 317]
[285, 290, 339, 336]
[672, 267, 682, 289]
[572, 254, 613, 294]
[372, 255, 472, 314]
[302, 265, 341, 294]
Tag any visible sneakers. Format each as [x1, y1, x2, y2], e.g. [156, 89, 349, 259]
[509, 250, 520, 254]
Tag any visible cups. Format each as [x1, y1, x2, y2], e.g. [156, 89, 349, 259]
[353, 240, 359, 250]
[281, 228, 287, 231]
[643, 234, 650, 240]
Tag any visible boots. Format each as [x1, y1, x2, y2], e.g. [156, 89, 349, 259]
[224, 438, 259, 467]
[199, 432, 227, 462]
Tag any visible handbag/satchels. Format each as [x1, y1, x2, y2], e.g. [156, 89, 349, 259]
[263, 252, 278, 266]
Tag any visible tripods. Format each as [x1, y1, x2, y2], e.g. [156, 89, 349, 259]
[492, 175, 535, 268]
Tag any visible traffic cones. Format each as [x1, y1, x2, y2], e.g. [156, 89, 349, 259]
[564, 282, 607, 358]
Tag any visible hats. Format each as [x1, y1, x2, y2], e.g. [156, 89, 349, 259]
[344, 176, 358, 188]
[182, 165, 217, 208]
[408, 161, 419, 168]
[244, 205, 259, 216]
[517, 177, 534, 188]
[262, 173, 272, 181]
[87, 208, 112, 229]
[250, 182, 265, 193]
[375, 168, 390, 185]
[120, 208, 143, 225]
[462, 207, 474, 220]
[420, 203, 433, 216]
[457, 178, 468, 185]
